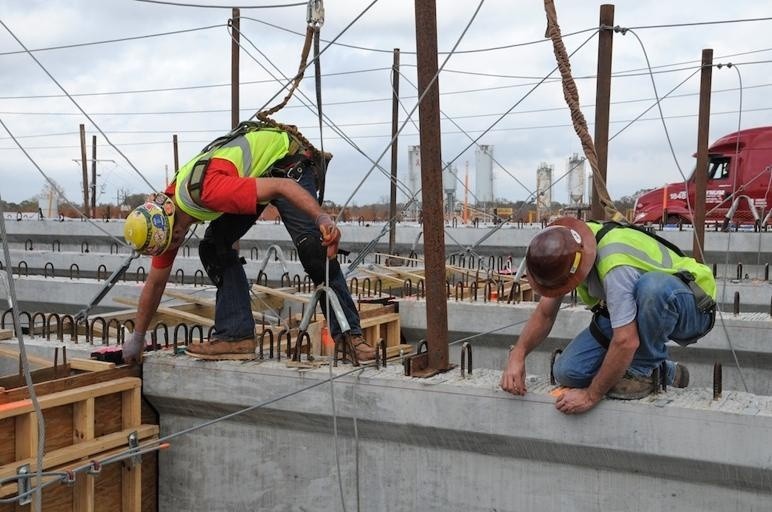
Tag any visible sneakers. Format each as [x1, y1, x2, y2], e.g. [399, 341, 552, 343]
[185, 337, 257, 361]
[608, 374, 655, 399]
[674, 364, 689, 388]
[335, 334, 382, 364]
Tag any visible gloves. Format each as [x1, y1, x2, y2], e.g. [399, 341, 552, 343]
[122, 328, 145, 365]
[315, 212, 340, 260]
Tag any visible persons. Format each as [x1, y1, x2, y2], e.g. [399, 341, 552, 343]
[497, 214, 718, 415]
[120, 121, 383, 367]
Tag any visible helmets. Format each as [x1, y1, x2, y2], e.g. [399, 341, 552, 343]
[124, 193, 175, 256]
[525, 216, 597, 298]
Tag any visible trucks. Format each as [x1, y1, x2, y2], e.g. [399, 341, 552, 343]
[627, 124, 772, 229]
